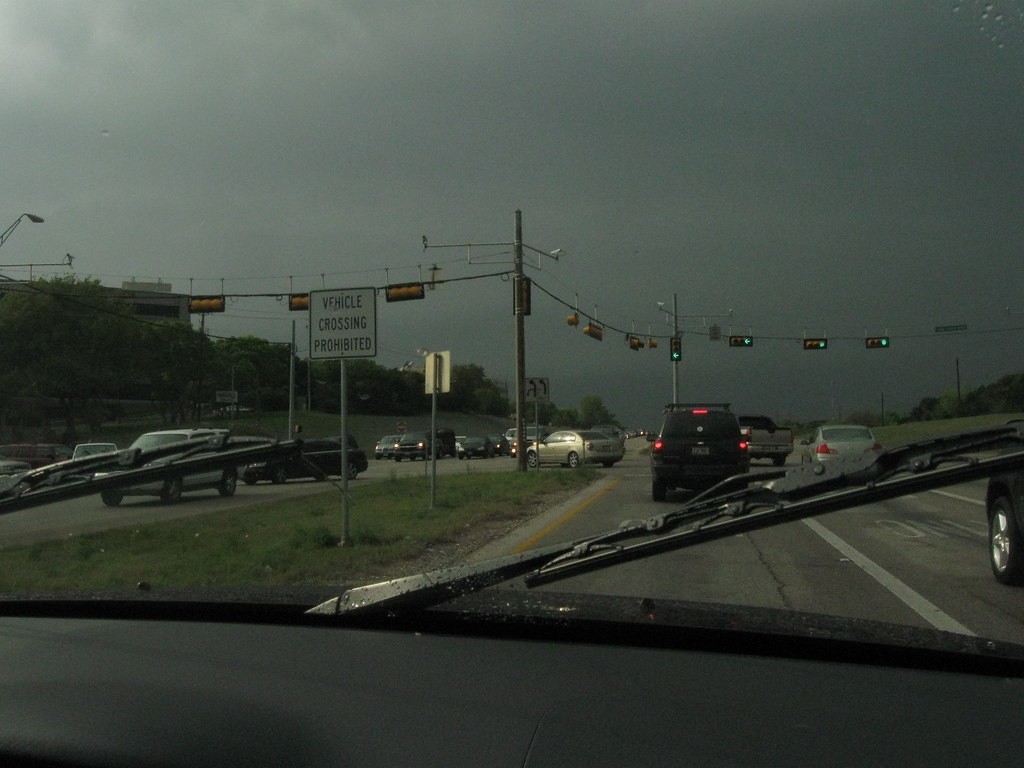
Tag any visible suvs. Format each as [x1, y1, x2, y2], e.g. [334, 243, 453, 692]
[242, 434, 369, 485]
[644, 401, 752, 503]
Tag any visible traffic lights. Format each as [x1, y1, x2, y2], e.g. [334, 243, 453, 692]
[730, 336, 752, 347]
[567, 312, 579, 328]
[804, 338, 828, 350]
[629, 337, 644, 352]
[583, 322, 603, 341]
[649, 338, 657, 349]
[670, 337, 682, 361]
[865, 337, 889, 349]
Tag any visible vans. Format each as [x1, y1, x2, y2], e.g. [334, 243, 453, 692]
[0, 443, 74, 480]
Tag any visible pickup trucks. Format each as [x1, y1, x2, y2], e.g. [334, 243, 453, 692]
[94, 426, 290, 506]
[735, 414, 795, 465]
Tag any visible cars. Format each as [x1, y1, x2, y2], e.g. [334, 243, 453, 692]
[72, 443, 120, 478]
[0, 460, 35, 484]
[526, 428, 626, 469]
[984, 414, 1024, 586]
[372, 427, 549, 461]
[798, 425, 885, 474]
[620, 428, 649, 440]
